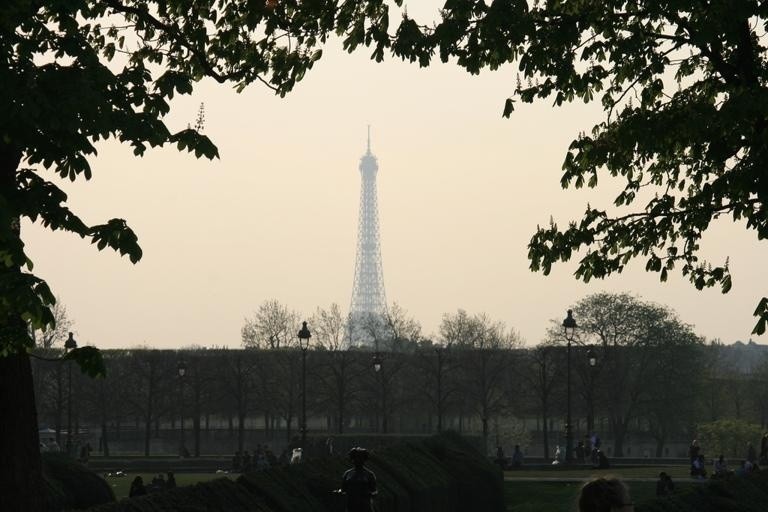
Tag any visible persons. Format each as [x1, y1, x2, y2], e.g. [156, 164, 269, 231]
[495, 447, 505, 461]
[36, 436, 94, 461]
[655, 441, 768, 495]
[511, 444, 523, 469]
[573, 432, 608, 470]
[127, 471, 176, 498]
[340, 445, 377, 511]
[576, 475, 634, 511]
[230, 442, 296, 474]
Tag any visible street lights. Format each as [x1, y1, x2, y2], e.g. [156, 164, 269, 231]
[177, 358, 187, 457]
[562, 309, 578, 463]
[586, 344, 597, 435]
[370, 351, 383, 432]
[63, 332, 77, 462]
[298, 321, 312, 443]
